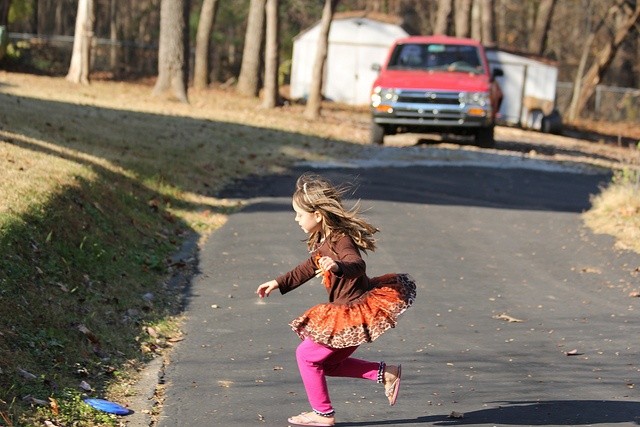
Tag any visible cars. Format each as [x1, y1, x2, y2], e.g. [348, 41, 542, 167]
[370, 36, 503, 146]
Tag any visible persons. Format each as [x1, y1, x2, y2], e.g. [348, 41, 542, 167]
[255, 174, 416, 427]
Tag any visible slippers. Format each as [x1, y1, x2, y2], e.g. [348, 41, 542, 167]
[384, 363, 402, 405]
[287, 411, 335, 427]
